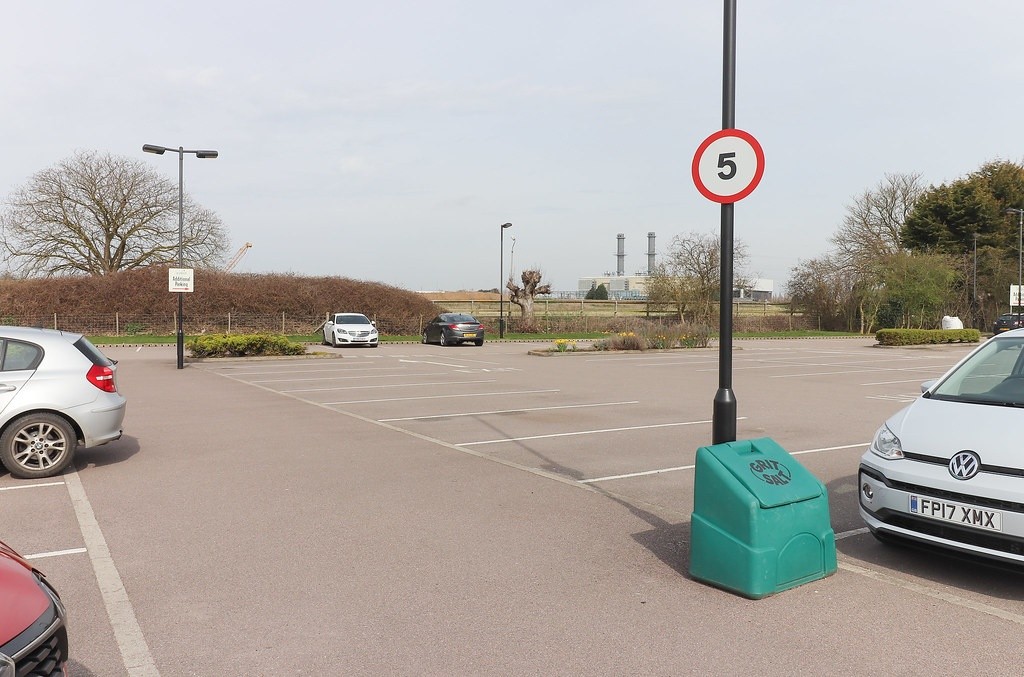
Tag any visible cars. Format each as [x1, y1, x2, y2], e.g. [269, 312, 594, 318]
[0, 325, 127, 479]
[422, 313, 484, 346]
[0, 540, 70, 677]
[858, 326, 1024, 577]
[322, 313, 378, 348]
[993, 313, 1024, 334]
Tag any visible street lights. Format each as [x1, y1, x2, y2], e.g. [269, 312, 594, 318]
[500, 222, 513, 338]
[142, 143, 219, 368]
[1006, 208, 1023, 326]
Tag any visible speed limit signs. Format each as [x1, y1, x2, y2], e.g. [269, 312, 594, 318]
[691, 129, 765, 204]
